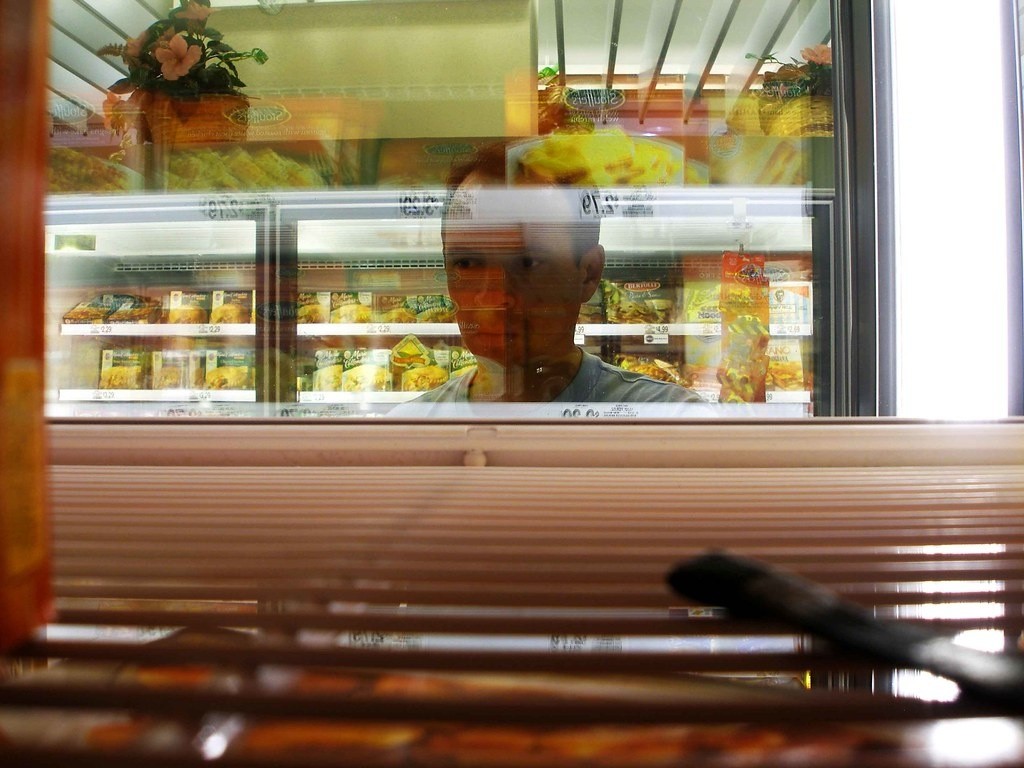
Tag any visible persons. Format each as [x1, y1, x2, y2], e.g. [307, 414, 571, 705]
[382, 135, 710, 408]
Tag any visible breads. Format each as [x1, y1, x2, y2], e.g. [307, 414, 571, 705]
[514, 128, 674, 188]
[167, 303, 454, 323]
[97, 364, 478, 394]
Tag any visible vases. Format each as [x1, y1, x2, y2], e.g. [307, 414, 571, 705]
[145, 94, 250, 143]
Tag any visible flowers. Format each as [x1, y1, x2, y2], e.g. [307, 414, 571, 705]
[95, 0, 269, 137]
[745, 44, 832, 130]
